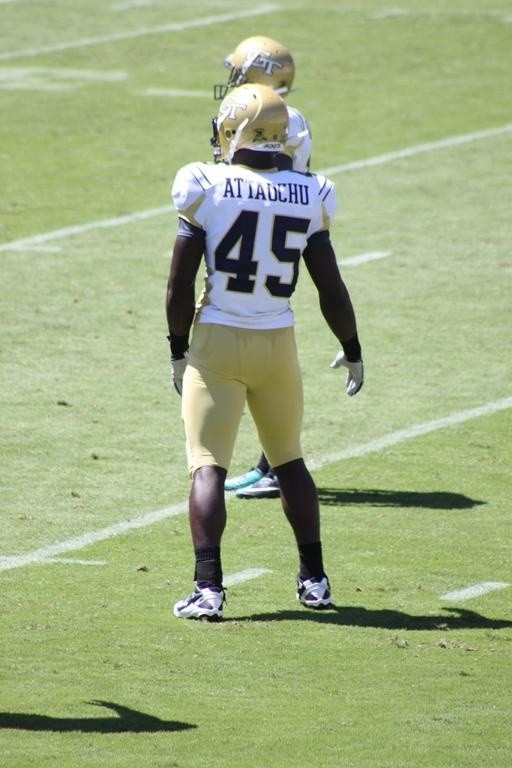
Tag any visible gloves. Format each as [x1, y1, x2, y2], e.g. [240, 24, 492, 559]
[330, 351, 364, 396]
[171, 347, 189, 396]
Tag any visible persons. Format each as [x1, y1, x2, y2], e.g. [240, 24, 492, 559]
[209, 33, 316, 502]
[161, 82, 367, 624]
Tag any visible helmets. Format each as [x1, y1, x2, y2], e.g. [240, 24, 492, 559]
[210, 82, 290, 168]
[221, 35, 296, 96]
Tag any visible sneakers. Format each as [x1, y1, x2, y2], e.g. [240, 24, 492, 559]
[224, 467, 264, 491]
[296, 573, 332, 608]
[173, 581, 226, 621]
[235, 472, 280, 498]
